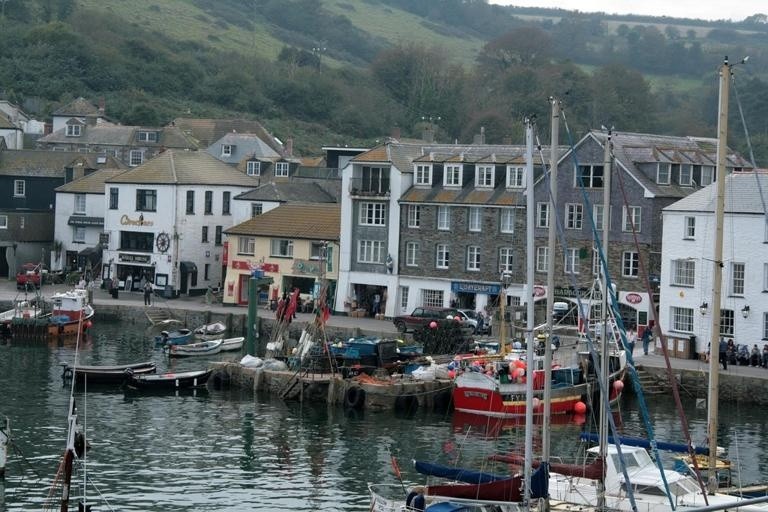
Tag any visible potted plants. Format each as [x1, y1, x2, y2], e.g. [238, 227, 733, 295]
[350, 187, 389, 196]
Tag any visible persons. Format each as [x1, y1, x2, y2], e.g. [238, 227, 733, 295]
[474, 311, 484, 336]
[301, 295, 311, 312]
[625, 328, 638, 355]
[143, 283, 153, 305]
[447, 311, 463, 322]
[111, 275, 120, 298]
[371, 291, 381, 313]
[640, 324, 654, 356]
[704, 336, 768, 369]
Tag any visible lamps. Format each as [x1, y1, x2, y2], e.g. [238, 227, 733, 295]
[700, 301, 707, 316]
[741, 305, 750, 319]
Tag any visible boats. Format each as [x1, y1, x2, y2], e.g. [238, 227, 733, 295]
[195, 320, 226, 341]
[0, 248, 93, 340]
[154, 328, 192, 346]
[164, 338, 223, 356]
[123, 367, 212, 391]
[61, 359, 156, 387]
